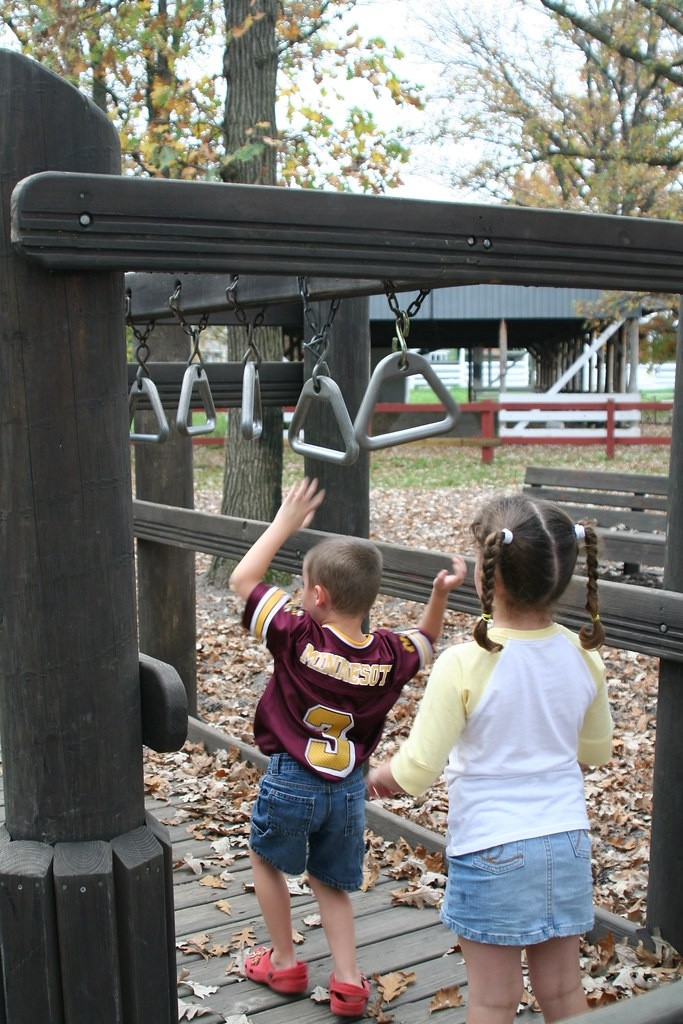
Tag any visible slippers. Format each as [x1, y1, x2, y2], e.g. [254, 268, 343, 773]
[244, 947, 308, 993]
[328, 971, 370, 1015]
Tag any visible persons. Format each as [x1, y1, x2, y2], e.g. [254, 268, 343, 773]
[226, 474, 468, 1015]
[362, 494, 616, 1024]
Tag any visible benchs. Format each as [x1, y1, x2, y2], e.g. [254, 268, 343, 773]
[522, 466, 669, 574]
